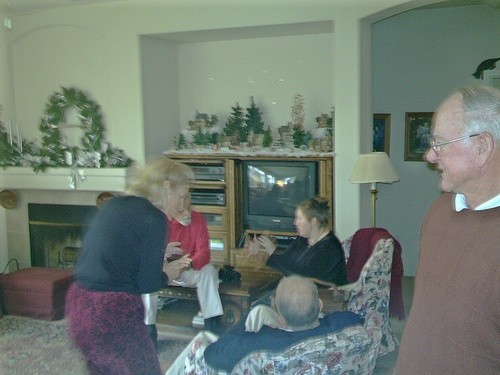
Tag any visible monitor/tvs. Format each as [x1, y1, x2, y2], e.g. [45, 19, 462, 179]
[242, 159, 319, 233]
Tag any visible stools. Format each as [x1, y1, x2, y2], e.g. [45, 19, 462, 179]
[0, 267, 77, 322]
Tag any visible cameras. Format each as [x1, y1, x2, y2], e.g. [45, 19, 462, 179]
[219, 266, 241, 283]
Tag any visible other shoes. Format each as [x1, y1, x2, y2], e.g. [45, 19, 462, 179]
[149, 326, 157, 346]
[203, 324, 224, 337]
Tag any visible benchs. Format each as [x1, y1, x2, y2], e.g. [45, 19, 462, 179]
[158, 271, 281, 326]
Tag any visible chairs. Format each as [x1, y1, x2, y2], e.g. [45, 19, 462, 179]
[164, 311, 382, 375]
[324, 226, 406, 360]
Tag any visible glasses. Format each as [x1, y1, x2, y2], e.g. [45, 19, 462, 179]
[429, 134, 478, 154]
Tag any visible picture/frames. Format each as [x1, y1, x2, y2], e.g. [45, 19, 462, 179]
[372, 113, 390, 157]
[404, 112, 434, 161]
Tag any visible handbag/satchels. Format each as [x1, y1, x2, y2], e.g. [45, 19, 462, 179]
[0, 258, 20, 317]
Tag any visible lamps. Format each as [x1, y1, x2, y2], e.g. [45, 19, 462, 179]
[350, 152, 400, 227]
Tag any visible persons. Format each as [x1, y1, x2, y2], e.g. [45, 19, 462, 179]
[393, 85, 500, 375]
[373, 125, 384, 149]
[143, 205, 225, 350]
[243, 195, 349, 307]
[204, 273, 364, 372]
[415, 120, 430, 151]
[65, 158, 194, 375]
